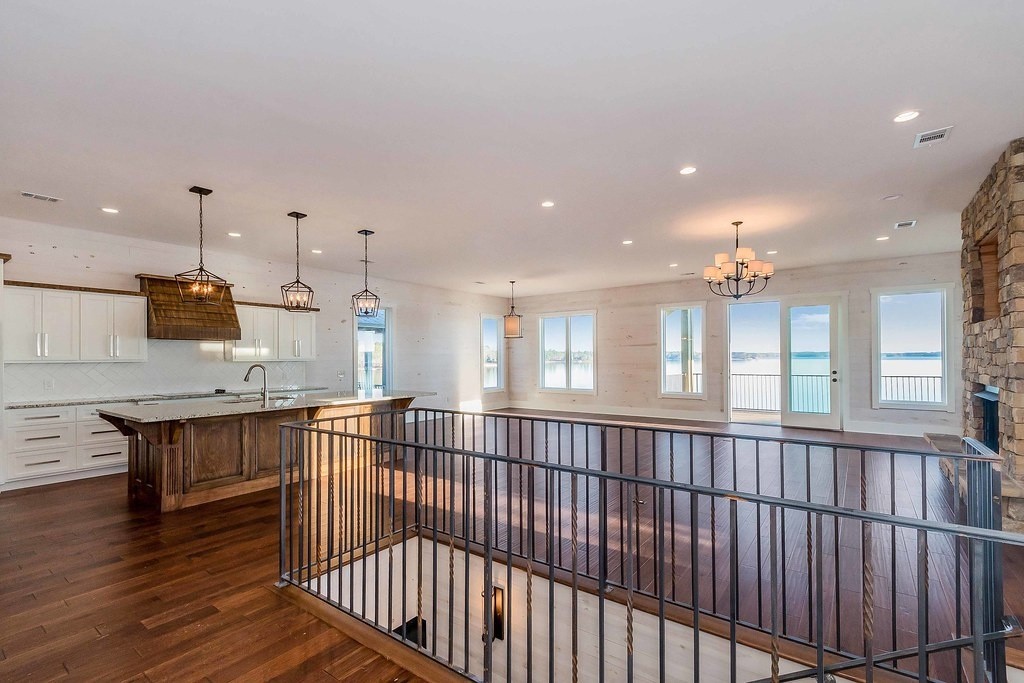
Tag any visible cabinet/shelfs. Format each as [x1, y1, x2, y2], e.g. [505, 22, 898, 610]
[79, 286, 148, 363]
[2, 280, 81, 364]
[76, 401, 138, 480]
[224, 301, 279, 362]
[278, 305, 320, 362]
[2, 406, 76, 492]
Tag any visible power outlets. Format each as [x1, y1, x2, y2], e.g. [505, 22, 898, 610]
[42, 377, 54, 391]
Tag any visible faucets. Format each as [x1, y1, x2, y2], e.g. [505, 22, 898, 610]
[244, 363, 269, 409]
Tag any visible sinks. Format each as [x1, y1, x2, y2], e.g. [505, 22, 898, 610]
[223, 395, 299, 408]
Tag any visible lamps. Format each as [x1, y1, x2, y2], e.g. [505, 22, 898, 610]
[503, 280, 524, 338]
[175, 185, 228, 307]
[352, 229, 381, 318]
[480, 586, 505, 644]
[281, 211, 314, 313]
[703, 221, 774, 300]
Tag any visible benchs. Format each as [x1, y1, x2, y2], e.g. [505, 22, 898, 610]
[923, 432, 1023, 513]
